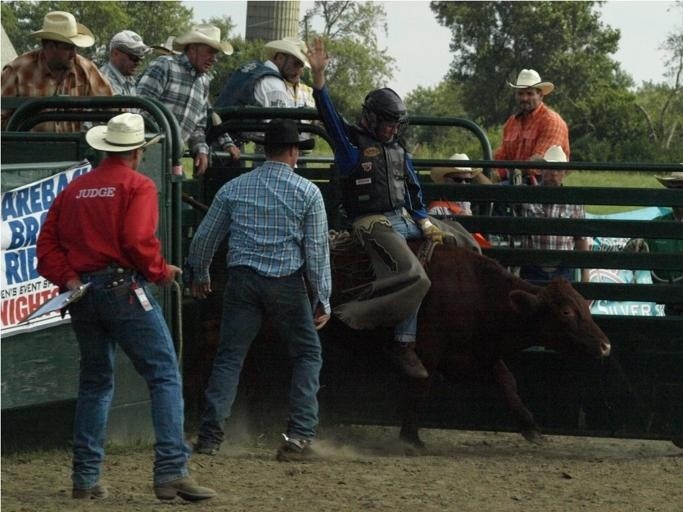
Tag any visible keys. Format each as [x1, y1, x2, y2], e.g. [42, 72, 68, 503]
[129, 275, 139, 305]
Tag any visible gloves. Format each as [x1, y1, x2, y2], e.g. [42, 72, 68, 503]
[418, 220, 452, 246]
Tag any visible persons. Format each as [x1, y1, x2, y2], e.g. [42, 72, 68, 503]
[36, 112, 217, 501]
[137, 23, 233, 173]
[515, 144, 589, 282]
[624, 171, 683, 317]
[427, 154, 491, 249]
[83, 30, 151, 129]
[207, 35, 317, 171]
[153, 35, 240, 160]
[4, 11, 126, 130]
[302, 34, 482, 379]
[189, 117, 332, 462]
[491, 68, 570, 186]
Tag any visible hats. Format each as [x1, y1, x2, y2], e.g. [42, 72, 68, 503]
[27, 10, 96, 48]
[110, 30, 155, 60]
[655, 164, 682, 188]
[528, 144, 575, 177]
[152, 34, 183, 55]
[85, 112, 167, 153]
[261, 35, 311, 69]
[251, 115, 316, 150]
[172, 23, 234, 56]
[430, 153, 483, 185]
[505, 67, 555, 95]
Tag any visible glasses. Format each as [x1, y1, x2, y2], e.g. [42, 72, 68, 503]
[121, 52, 138, 61]
[448, 176, 472, 183]
[56, 42, 76, 50]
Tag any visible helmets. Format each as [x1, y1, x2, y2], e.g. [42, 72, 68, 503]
[361, 88, 408, 146]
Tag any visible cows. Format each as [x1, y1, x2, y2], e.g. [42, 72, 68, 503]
[182, 192, 612, 447]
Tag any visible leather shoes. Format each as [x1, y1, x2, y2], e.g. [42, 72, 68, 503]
[383, 345, 429, 379]
[197, 440, 219, 455]
[276, 440, 323, 461]
[155, 474, 218, 502]
[72, 483, 110, 501]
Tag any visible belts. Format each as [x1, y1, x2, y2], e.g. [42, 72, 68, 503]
[539, 266, 563, 272]
